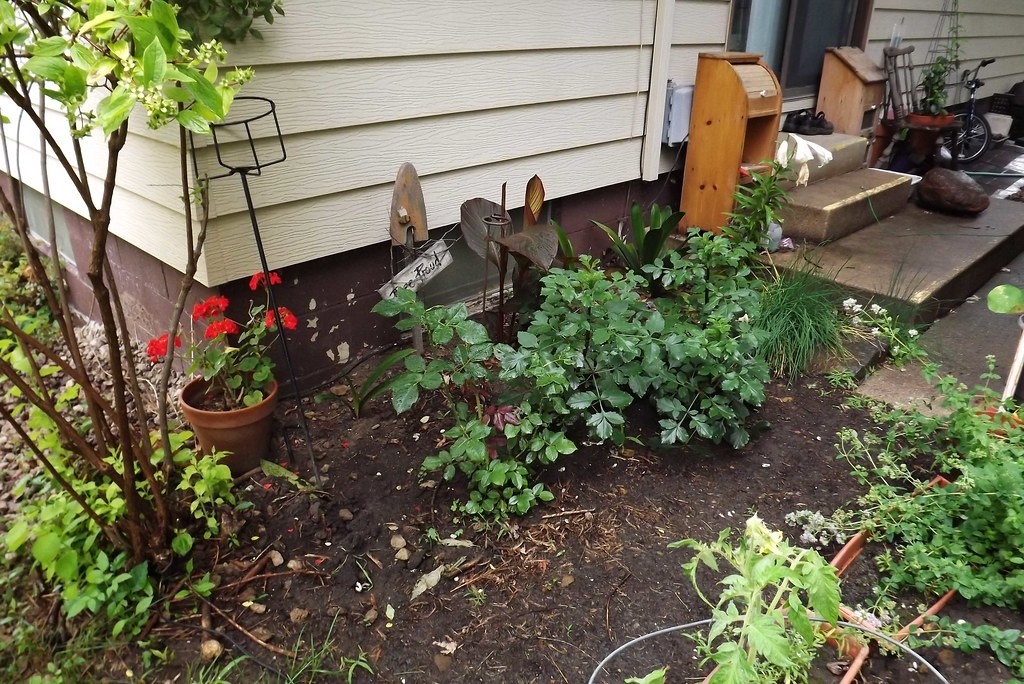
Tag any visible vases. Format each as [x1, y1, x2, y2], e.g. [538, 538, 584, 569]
[179, 365, 278, 471]
[869, 124, 889, 167]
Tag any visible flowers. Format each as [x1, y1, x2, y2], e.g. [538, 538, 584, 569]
[147, 271, 297, 409]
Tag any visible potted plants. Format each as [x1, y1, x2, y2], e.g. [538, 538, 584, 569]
[910, 12, 965, 155]
[726, 159, 798, 252]
[672, 298, 1024, 684]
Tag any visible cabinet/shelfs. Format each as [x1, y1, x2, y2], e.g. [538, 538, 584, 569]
[676, 50, 784, 253]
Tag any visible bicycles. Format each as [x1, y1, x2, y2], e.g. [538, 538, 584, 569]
[940, 57, 996, 164]
